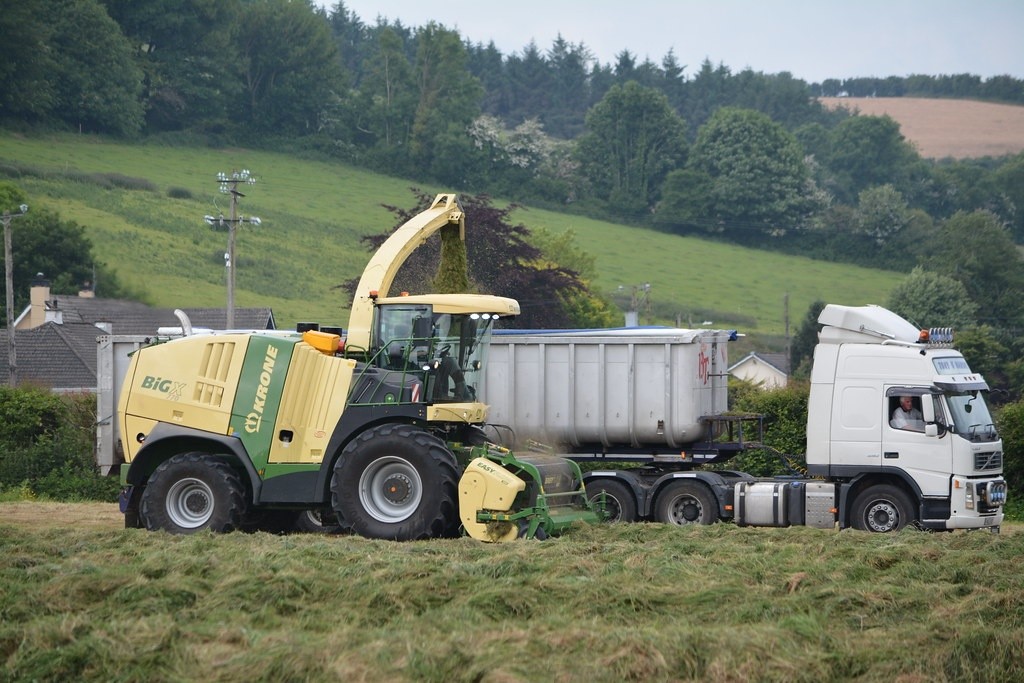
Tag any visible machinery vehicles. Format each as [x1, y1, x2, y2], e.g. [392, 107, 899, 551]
[118, 194, 599, 540]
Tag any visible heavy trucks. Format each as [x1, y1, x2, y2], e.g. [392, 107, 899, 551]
[95, 304, 1007, 534]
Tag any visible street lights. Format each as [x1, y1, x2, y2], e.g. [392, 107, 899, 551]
[206, 170, 261, 328]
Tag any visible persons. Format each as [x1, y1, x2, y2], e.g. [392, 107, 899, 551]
[891, 395, 924, 432]
